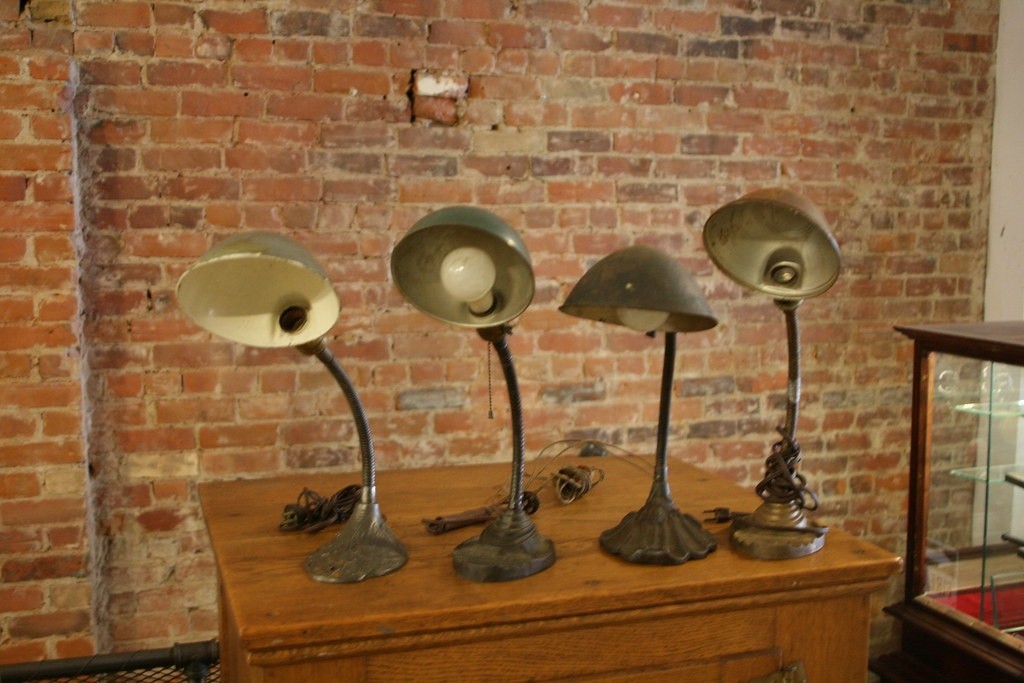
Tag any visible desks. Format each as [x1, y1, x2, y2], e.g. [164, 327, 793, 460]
[196, 454, 903, 683]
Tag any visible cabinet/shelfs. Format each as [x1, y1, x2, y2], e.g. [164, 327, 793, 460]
[868, 317, 1023, 683]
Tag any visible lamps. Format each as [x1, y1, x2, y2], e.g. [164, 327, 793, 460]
[390, 205, 554, 587]
[559, 247, 718, 566]
[703, 184, 840, 559]
[171, 233, 406, 584]
